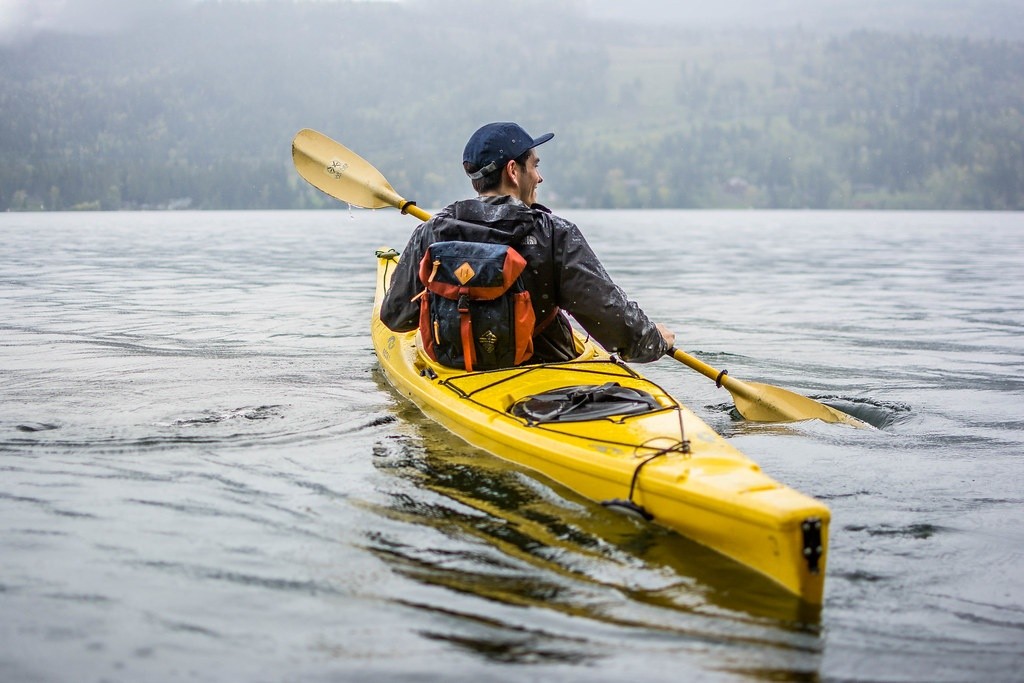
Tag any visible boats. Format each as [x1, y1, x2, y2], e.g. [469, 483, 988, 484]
[371, 245, 834, 607]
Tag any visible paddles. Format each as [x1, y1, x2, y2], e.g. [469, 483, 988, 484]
[289, 124, 880, 433]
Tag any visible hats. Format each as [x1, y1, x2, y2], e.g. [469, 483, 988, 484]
[463, 121, 554, 180]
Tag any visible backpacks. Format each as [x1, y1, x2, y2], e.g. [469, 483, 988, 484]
[419, 240, 558, 374]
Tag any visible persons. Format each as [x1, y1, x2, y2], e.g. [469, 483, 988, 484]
[380, 122, 677, 371]
